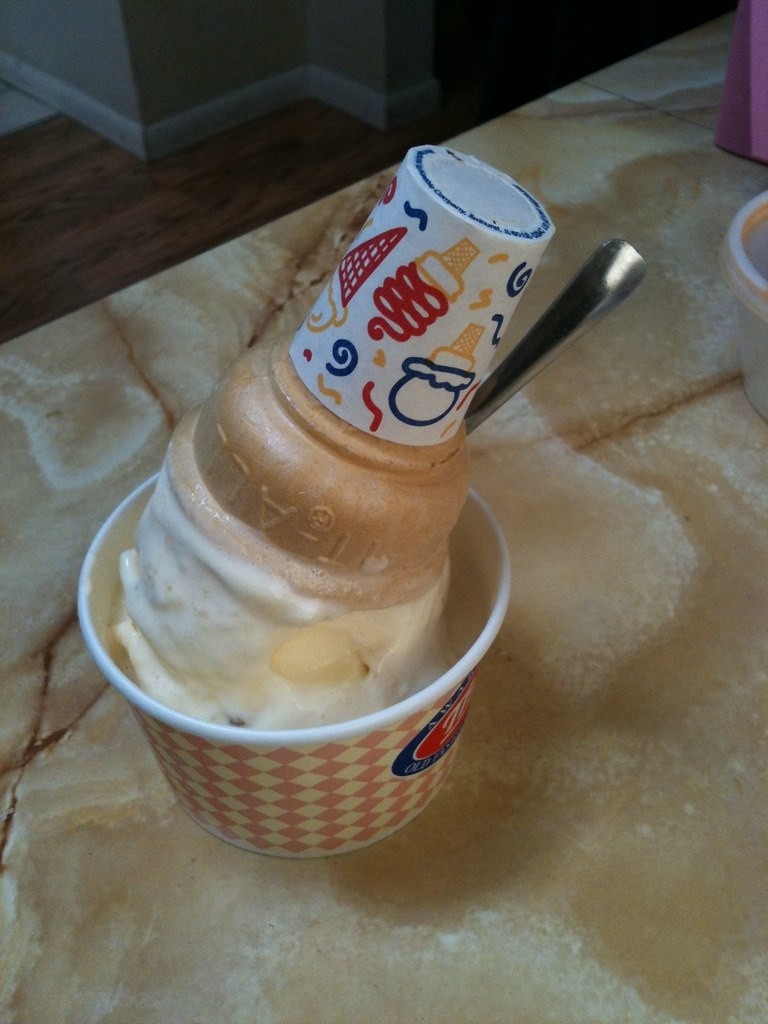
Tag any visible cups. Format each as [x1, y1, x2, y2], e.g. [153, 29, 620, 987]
[78, 464, 513, 857]
[717, 187, 768, 418]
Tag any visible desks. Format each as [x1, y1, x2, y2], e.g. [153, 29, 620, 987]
[0, 11, 768, 1024]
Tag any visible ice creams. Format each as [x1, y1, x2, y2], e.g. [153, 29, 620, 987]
[97, 146, 556, 731]
